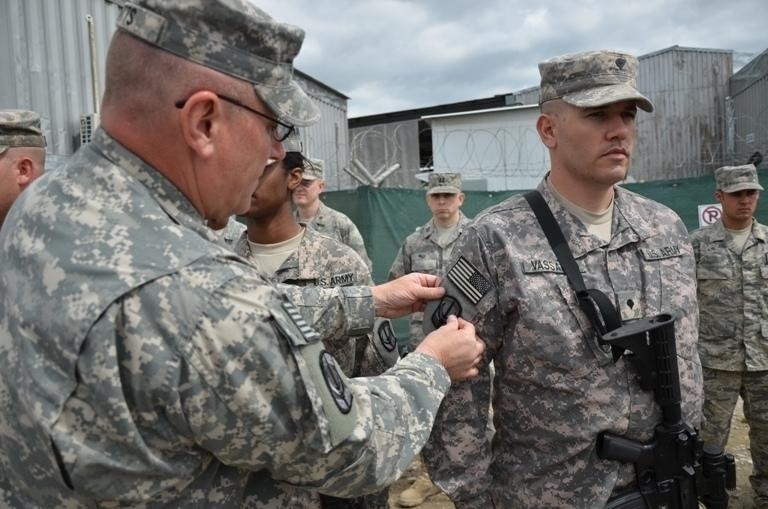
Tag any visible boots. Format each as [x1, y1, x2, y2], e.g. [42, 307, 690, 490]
[400, 454, 442, 507]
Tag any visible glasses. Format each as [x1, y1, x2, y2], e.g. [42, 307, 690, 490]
[173, 93, 295, 143]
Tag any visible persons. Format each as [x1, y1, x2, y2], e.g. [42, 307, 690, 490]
[689, 164, 767, 509]
[289, 157, 374, 276]
[0, 106, 48, 228]
[225, 122, 372, 384]
[386, 168, 493, 507]
[418, 49, 707, 508]
[0, 0, 484, 508]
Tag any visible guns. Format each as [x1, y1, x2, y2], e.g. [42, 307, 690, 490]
[597, 313, 737, 508]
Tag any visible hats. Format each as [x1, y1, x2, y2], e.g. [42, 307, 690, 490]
[114, 0, 321, 128]
[301, 156, 326, 181]
[538, 48, 653, 116]
[424, 166, 462, 197]
[0, 106, 48, 156]
[714, 163, 764, 195]
